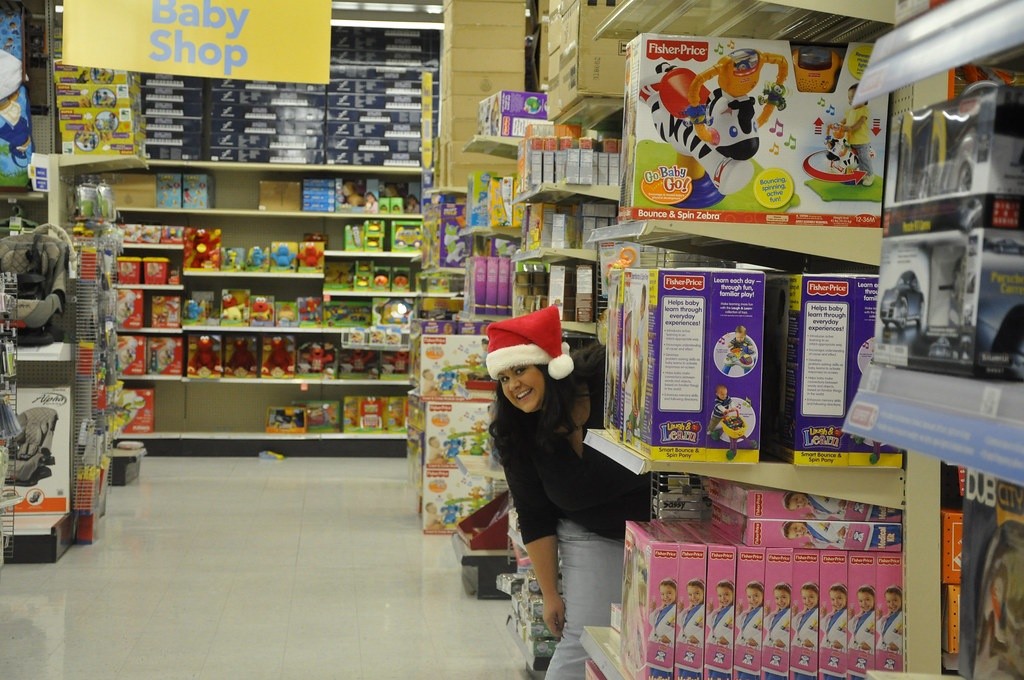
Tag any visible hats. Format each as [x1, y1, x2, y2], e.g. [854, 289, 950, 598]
[486, 305, 574, 380]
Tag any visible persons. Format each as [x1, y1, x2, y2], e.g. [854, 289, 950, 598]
[707, 385, 733, 437]
[781, 491, 902, 550]
[486, 305, 710, 680]
[723, 326, 757, 376]
[647, 578, 904, 680]
[839, 83, 875, 186]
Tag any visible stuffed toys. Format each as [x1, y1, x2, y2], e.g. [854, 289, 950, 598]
[301, 343, 334, 375]
[190, 228, 222, 268]
[188, 335, 295, 378]
[221, 294, 248, 322]
[250, 296, 271, 320]
[187, 300, 204, 320]
[249, 242, 322, 267]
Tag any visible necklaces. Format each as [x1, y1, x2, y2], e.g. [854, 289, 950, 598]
[574, 412, 589, 430]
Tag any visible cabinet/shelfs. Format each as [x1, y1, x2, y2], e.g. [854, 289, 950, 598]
[2, 188, 71, 563]
[512, 179, 621, 342]
[455, 134, 564, 329]
[577, 1, 909, 679]
[101, 152, 437, 460]
[436, 181, 471, 278]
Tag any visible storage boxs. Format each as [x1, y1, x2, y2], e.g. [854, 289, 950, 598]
[0, 0, 1024, 679]
